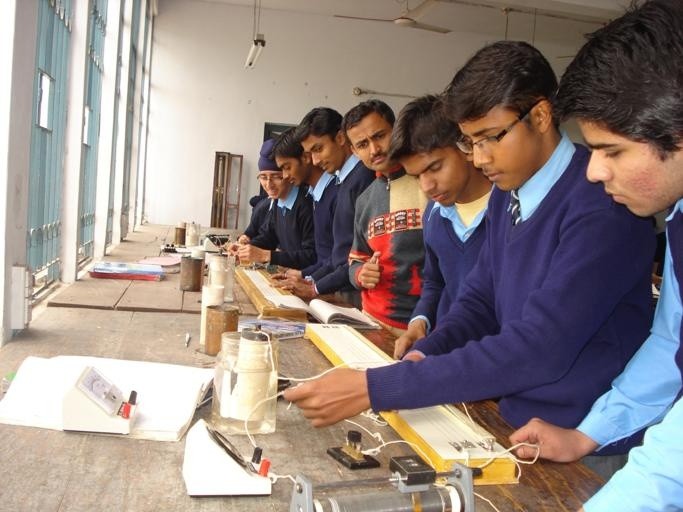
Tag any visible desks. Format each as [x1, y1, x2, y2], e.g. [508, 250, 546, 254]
[0, 306, 609, 512]
[47, 222, 392, 332]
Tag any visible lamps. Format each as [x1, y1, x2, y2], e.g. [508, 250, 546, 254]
[245, 0, 265, 70]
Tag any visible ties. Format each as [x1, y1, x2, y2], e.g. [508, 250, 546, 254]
[505, 189, 524, 226]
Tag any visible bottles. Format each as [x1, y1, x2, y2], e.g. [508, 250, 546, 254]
[188, 224, 199, 246]
[212, 331, 277, 435]
[207, 255, 235, 303]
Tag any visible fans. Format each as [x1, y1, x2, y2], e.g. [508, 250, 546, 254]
[333, 0, 453, 34]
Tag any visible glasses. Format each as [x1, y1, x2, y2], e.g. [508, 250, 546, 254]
[258, 174, 283, 181]
[454, 97, 548, 154]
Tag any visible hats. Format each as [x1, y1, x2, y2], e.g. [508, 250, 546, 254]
[257, 138, 286, 171]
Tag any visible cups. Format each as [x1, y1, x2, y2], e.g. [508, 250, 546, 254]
[239, 258, 255, 267]
[205, 304, 239, 356]
[174, 227, 186, 245]
[200, 284, 225, 345]
[179, 255, 204, 292]
[190, 248, 205, 260]
